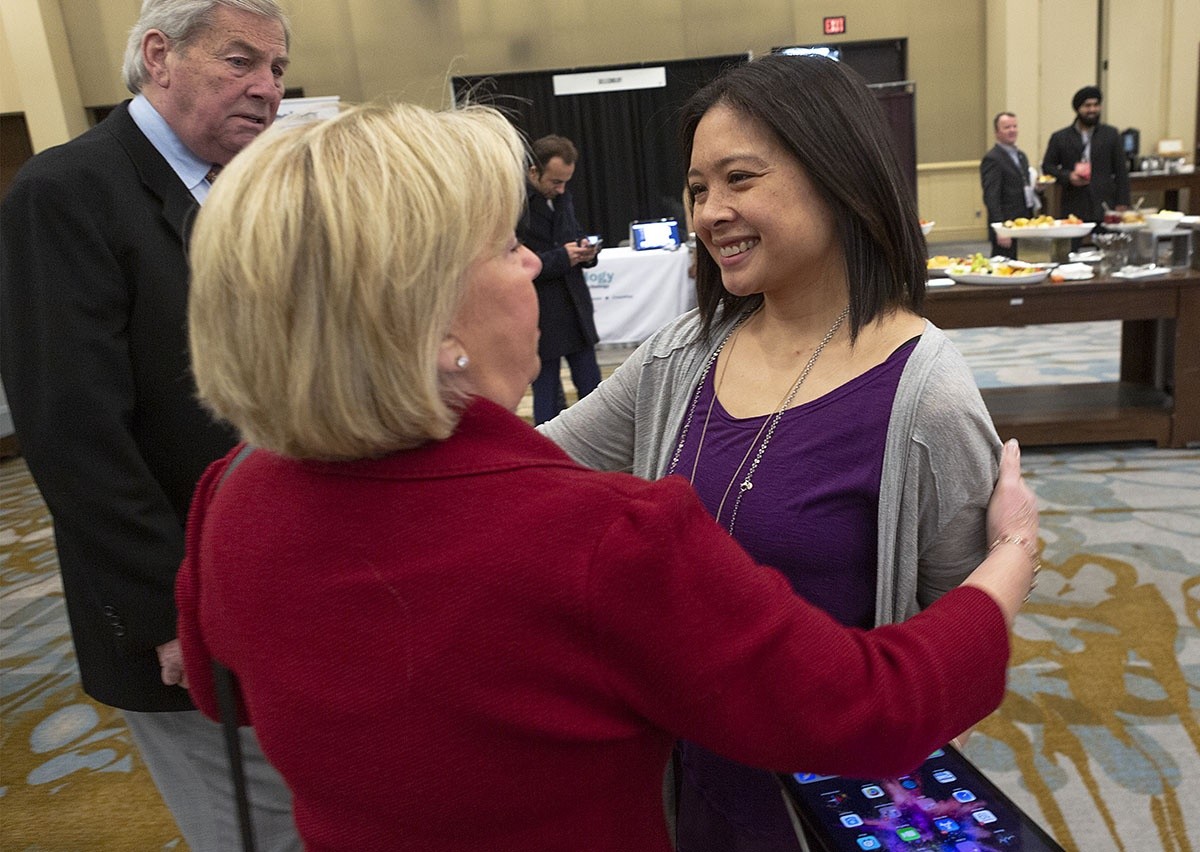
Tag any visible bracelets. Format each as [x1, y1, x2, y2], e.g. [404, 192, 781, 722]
[986, 532, 1041, 604]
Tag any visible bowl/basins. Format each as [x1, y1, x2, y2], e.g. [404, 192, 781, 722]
[1142, 213, 1185, 232]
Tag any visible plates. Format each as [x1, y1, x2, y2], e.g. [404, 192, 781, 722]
[1111, 267, 1172, 280]
[1100, 222, 1153, 232]
[919, 219, 1106, 286]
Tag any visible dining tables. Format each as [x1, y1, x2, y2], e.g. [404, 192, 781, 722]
[920, 263, 1200, 447]
[1036, 173, 1200, 215]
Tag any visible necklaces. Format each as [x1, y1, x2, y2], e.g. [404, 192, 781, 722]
[669, 304, 850, 538]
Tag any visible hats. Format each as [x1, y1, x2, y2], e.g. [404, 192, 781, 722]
[1073, 87, 1101, 110]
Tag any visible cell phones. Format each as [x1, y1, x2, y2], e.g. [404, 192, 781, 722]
[589, 238, 604, 248]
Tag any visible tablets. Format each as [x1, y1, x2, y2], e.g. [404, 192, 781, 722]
[776, 743, 1069, 852]
[629, 218, 681, 251]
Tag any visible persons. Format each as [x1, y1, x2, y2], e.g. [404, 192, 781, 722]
[516, 134, 604, 426]
[980, 113, 1045, 262]
[1, 0, 307, 852]
[531, 55, 1005, 852]
[173, 104, 1044, 852]
[1041, 86, 1132, 252]
